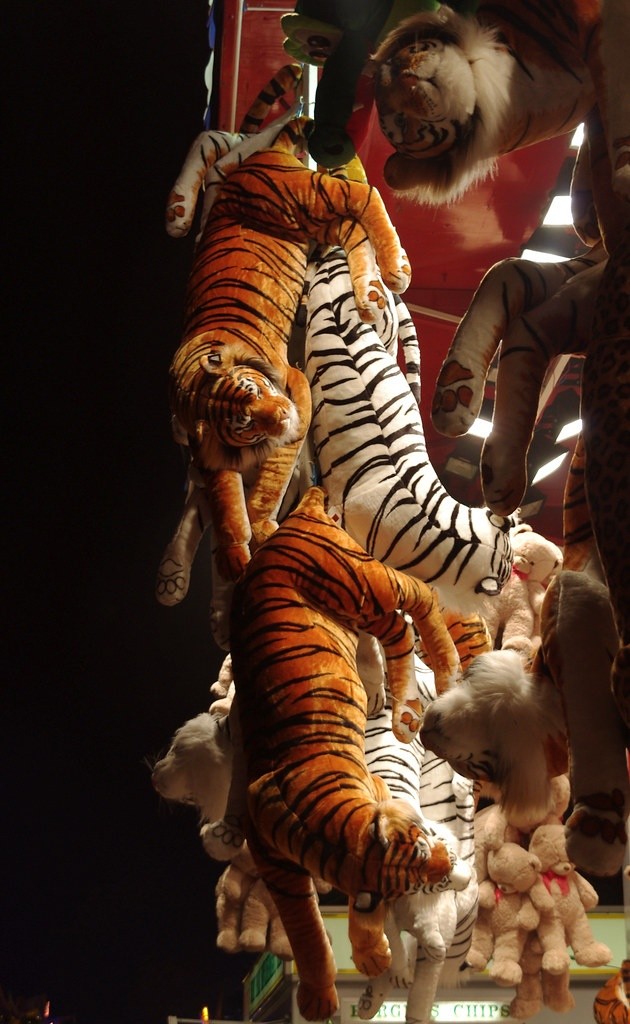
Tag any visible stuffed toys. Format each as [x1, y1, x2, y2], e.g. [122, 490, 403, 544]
[153, 0, 630, 1024]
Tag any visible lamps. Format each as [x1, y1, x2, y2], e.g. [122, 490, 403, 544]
[539, 157, 575, 228]
[444, 442, 481, 479]
[527, 431, 570, 487]
[466, 397, 494, 438]
[518, 227, 577, 263]
[542, 388, 583, 444]
[519, 488, 546, 520]
